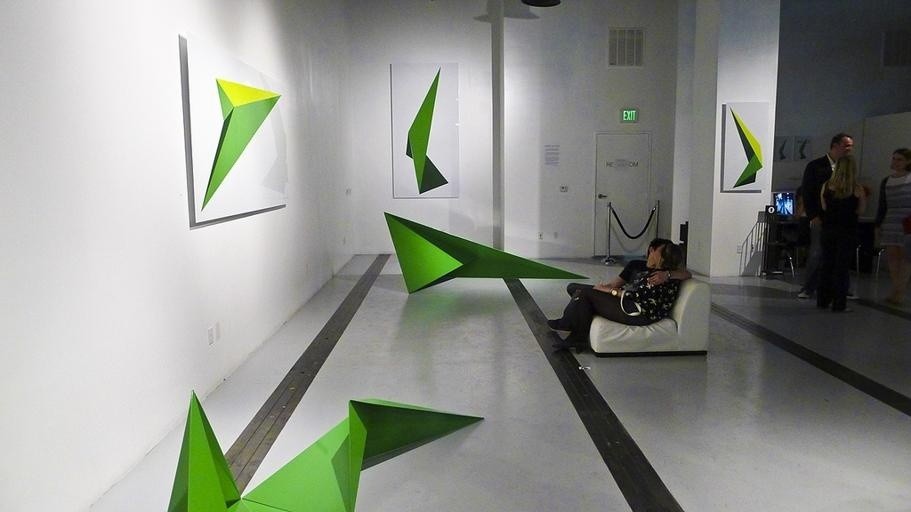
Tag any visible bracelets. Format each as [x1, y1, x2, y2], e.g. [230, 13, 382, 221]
[609, 287, 619, 297]
[667, 270, 673, 279]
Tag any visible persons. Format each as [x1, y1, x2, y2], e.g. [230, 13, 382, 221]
[872, 147, 911, 304]
[552, 241, 687, 355]
[816, 153, 868, 314]
[799, 132, 864, 300]
[796, 186, 813, 269]
[546, 238, 693, 332]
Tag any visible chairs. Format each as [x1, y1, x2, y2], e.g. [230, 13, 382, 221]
[765, 216, 812, 280]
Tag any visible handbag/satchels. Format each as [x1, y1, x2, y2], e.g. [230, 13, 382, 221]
[609, 287, 632, 299]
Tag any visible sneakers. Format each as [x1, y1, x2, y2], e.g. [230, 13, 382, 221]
[552, 333, 577, 347]
[832, 307, 853, 312]
[845, 293, 859, 299]
[548, 319, 574, 332]
[798, 288, 814, 298]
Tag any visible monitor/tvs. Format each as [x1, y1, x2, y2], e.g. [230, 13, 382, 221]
[772, 192, 794, 217]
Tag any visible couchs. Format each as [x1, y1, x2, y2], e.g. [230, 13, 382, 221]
[589, 278, 711, 358]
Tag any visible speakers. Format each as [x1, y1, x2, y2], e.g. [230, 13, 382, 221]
[766, 206, 777, 215]
